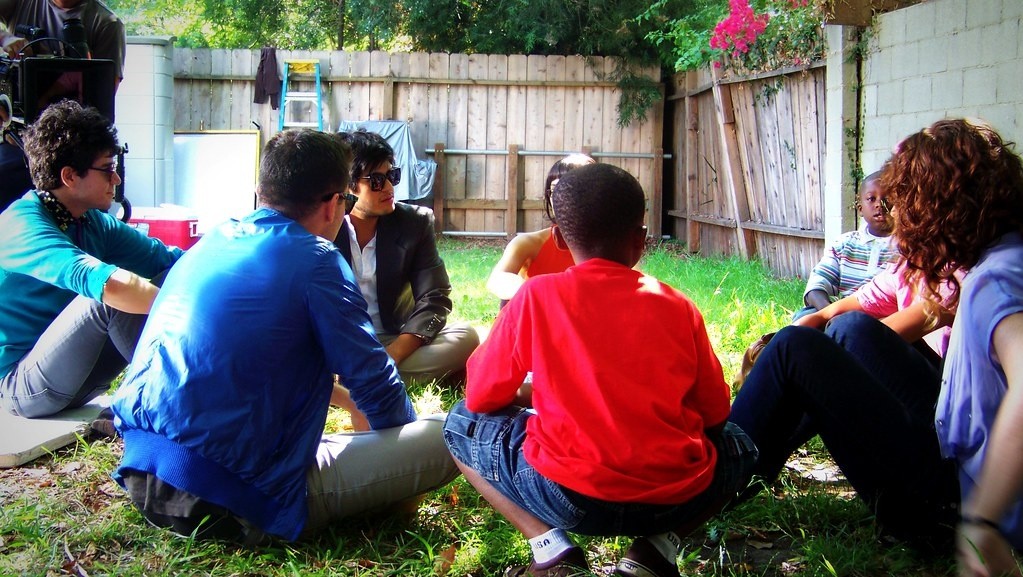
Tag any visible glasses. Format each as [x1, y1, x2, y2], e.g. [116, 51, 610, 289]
[88, 164, 115, 176]
[323, 192, 358, 215]
[881, 197, 893, 215]
[357, 167, 401, 192]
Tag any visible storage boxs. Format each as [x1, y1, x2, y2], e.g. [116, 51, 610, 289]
[117, 206, 200, 252]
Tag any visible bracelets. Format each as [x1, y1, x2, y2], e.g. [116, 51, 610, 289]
[959, 515, 1001, 534]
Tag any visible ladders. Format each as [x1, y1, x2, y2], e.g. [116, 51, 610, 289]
[279, 59, 323, 131]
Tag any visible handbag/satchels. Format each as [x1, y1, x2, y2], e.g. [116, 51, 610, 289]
[733, 332, 775, 392]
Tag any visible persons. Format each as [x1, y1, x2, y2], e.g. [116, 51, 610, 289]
[0, 0, 125, 214]
[0, 99, 187, 418]
[110, 128, 462, 547]
[333, 132, 481, 390]
[443, 164, 759, 577]
[729, 118, 1023, 577]
[486, 153, 596, 312]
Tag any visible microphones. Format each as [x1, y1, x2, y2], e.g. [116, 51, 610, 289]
[16, 24, 46, 40]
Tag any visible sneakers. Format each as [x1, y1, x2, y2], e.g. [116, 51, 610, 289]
[615, 537, 682, 577]
[503, 546, 592, 577]
